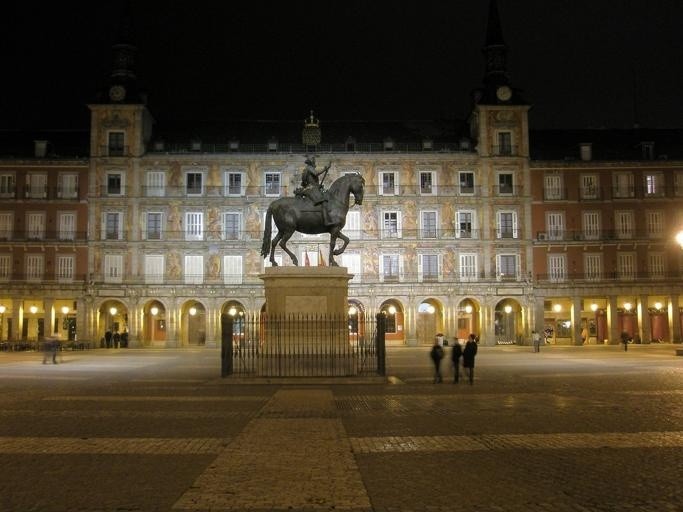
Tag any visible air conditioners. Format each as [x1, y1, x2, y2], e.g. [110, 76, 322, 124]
[573, 233, 583, 242]
[536, 232, 548, 241]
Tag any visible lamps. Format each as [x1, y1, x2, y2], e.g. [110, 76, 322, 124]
[624, 300, 632, 311]
[427, 298, 435, 314]
[504, 298, 513, 313]
[465, 298, 473, 314]
[590, 299, 598, 312]
[654, 299, 663, 310]
[348, 301, 357, 315]
[0, 298, 238, 320]
[553, 298, 562, 312]
[388, 301, 397, 315]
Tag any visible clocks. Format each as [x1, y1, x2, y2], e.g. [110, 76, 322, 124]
[495, 86, 511, 102]
[107, 85, 126, 102]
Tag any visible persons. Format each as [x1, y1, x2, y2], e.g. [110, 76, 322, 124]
[0, 324, 128, 364]
[530, 327, 633, 353]
[301, 156, 335, 226]
[429, 340, 444, 382]
[463, 334, 476, 387]
[451, 338, 463, 384]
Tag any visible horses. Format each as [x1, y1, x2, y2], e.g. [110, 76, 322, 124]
[260, 170, 365, 267]
